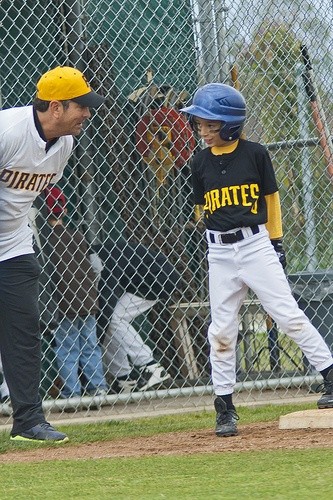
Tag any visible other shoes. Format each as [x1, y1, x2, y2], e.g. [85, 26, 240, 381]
[59, 395, 84, 412]
[0, 396, 13, 418]
[85, 389, 108, 408]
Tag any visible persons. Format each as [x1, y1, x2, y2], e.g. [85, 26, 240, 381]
[0, 183, 107, 416]
[179, 83, 333, 437]
[0, 66, 110, 444]
[88, 242, 177, 394]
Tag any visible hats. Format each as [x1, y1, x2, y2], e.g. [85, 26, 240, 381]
[36, 65, 106, 107]
[44, 185, 66, 213]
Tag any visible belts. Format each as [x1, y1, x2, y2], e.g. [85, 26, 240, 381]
[211, 224, 260, 243]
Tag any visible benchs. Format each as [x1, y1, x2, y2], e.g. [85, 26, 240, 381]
[167, 298, 267, 380]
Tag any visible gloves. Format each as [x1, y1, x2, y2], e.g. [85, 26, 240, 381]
[270, 240, 287, 269]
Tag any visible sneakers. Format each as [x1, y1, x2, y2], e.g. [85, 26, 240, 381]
[130, 363, 171, 392]
[107, 375, 137, 395]
[214, 397, 239, 436]
[315, 369, 333, 409]
[10, 422, 69, 444]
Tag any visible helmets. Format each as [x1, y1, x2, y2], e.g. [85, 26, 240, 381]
[179, 83, 247, 141]
